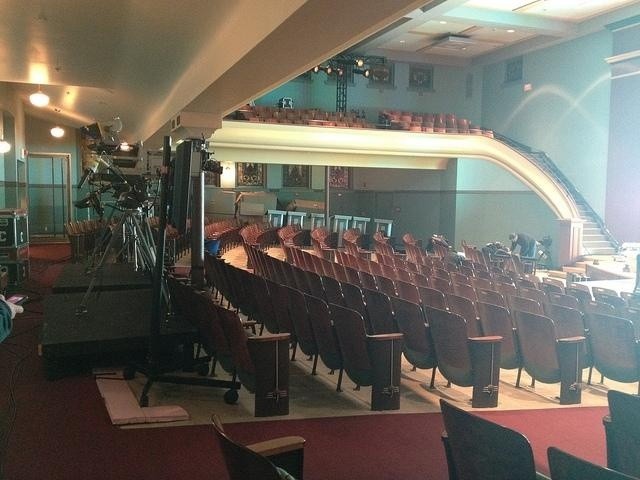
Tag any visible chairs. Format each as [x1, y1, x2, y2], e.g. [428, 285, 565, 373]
[235, 100, 494, 138]
[62, 215, 160, 265]
[160, 216, 639, 480]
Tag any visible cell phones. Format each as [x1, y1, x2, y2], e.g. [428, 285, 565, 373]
[6, 294, 29, 306]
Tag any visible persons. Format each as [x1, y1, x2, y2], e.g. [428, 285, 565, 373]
[0, 295, 24, 343]
[509, 232, 536, 258]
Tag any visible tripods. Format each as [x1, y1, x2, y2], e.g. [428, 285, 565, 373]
[77, 151, 175, 316]
[537, 248, 553, 270]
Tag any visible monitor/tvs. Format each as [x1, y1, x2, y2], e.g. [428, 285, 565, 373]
[168, 139, 193, 234]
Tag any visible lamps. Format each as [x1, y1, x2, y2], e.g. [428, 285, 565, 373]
[0, 132, 12, 155]
[303, 48, 372, 82]
[47, 124, 66, 140]
[27, 83, 51, 109]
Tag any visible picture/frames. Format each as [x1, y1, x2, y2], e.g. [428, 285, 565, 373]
[325, 166, 353, 193]
[281, 165, 312, 191]
[236, 162, 267, 190]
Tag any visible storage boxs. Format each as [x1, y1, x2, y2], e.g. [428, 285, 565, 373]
[200, 186, 327, 221]
